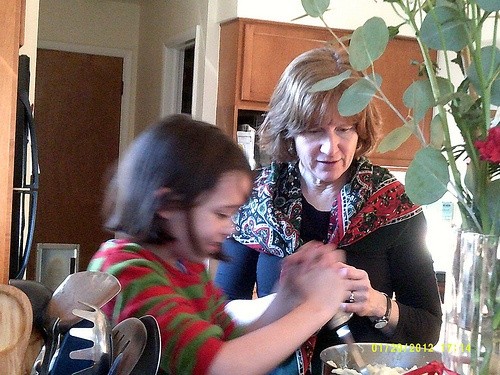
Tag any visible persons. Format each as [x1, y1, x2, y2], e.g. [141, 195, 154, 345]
[210, 47, 443, 375]
[85, 112, 353, 375]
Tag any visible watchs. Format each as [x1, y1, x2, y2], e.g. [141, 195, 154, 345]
[372, 291, 392, 329]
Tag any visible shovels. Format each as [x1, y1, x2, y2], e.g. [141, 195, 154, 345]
[39, 271, 122, 374]
[140, 315, 162, 374]
[111, 316, 147, 374]
[9, 279, 54, 343]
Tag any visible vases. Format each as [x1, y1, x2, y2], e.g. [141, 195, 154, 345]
[441, 229, 500, 375]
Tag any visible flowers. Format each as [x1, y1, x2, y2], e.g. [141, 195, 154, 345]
[291, 0, 500, 375]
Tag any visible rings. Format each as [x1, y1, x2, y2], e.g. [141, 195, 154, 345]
[350, 290, 355, 303]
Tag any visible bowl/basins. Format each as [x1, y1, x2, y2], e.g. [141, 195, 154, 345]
[320, 343, 444, 375]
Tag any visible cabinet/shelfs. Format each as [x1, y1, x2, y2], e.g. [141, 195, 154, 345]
[220, 17, 438, 168]
